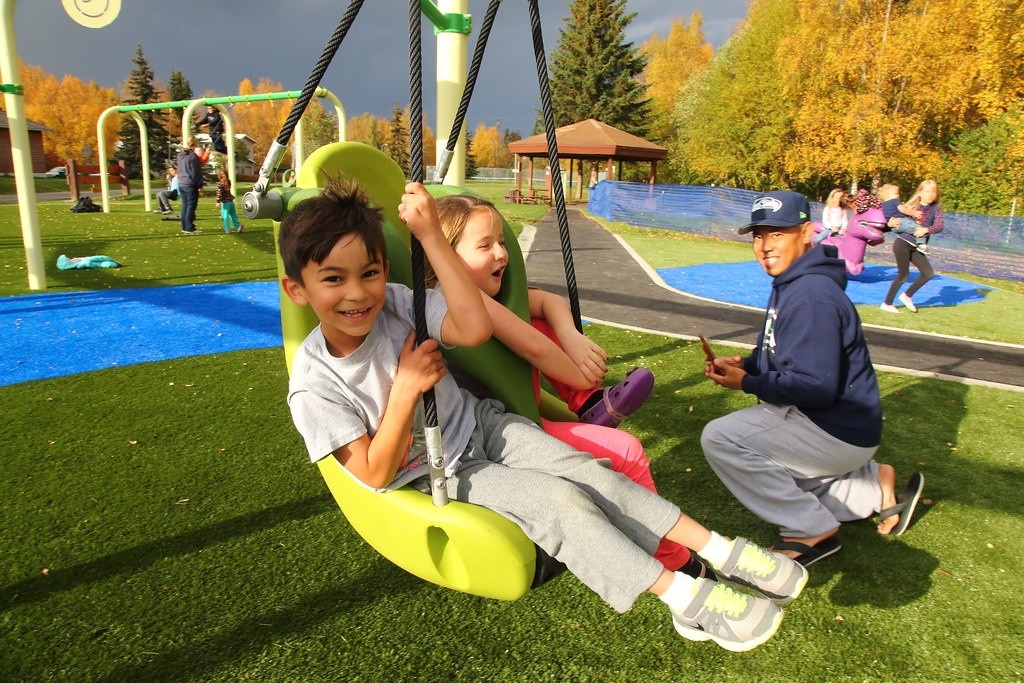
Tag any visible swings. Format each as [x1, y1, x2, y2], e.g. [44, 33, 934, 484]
[206, 101, 253, 148]
[163, 108, 179, 200]
[239, 0, 586, 595]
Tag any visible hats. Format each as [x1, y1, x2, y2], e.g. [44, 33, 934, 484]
[738, 191, 810, 235]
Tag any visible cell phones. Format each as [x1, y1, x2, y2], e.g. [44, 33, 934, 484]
[699, 334, 725, 375]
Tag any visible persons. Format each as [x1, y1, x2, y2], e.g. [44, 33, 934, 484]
[879, 180, 943, 314]
[423, 194, 718, 583]
[215, 170, 244, 234]
[192, 105, 226, 153]
[700, 192, 925, 565]
[278, 169, 809, 652]
[813, 189, 848, 245]
[878, 183, 931, 255]
[194, 145, 210, 164]
[176, 136, 204, 234]
[152, 167, 181, 215]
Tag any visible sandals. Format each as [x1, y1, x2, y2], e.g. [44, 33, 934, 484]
[579, 367, 655, 429]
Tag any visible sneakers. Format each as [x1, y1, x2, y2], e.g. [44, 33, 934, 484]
[673, 577, 784, 653]
[711, 536, 809, 606]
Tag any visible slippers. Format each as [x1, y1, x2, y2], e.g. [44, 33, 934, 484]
[877, 473, 924, 537]
[773, 535, 841, 567]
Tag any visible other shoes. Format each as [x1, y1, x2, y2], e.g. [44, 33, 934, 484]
[181, 229, 203, 233]
[899, 293, 918, 312]
[917, 246, 930, 255]
[880, 303, 899, 314]
[237, 225, 244, 233]
[153, 208, 163, 214]
[162, 209, 174, 215]
[225, 231, 231, 234]
